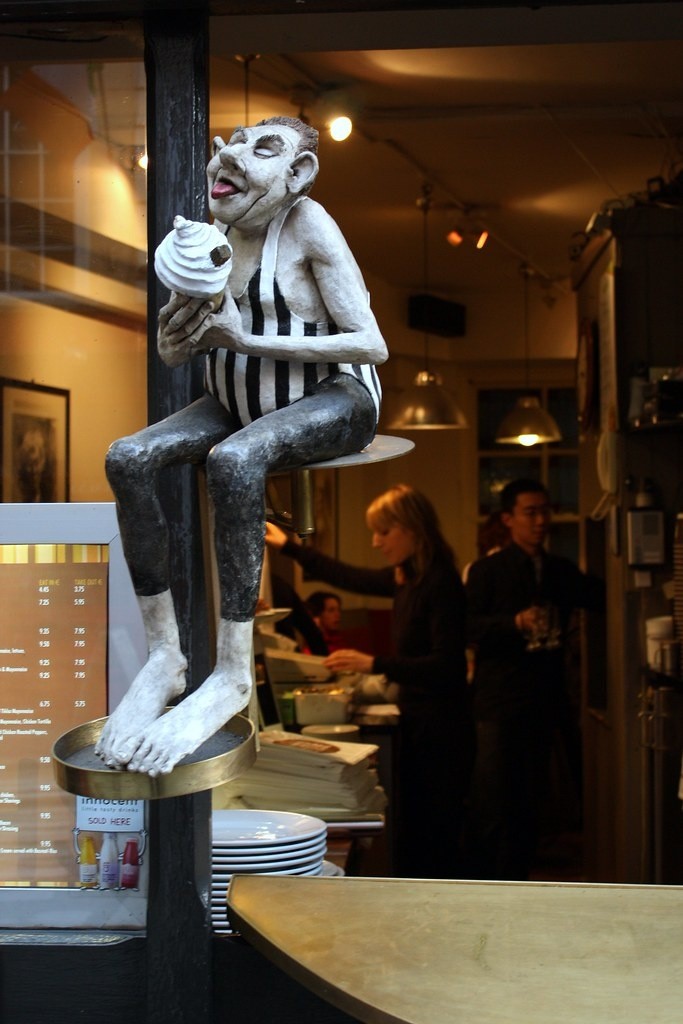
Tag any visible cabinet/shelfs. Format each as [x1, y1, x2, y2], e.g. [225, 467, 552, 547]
[571, 203, 683, 884]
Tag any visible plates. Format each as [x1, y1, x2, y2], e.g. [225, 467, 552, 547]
[210, 806, 346, 934]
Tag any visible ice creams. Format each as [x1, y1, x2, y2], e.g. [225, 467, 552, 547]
[153, 215, 233, 313]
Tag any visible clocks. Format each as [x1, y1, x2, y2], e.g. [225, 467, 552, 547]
[574, 320, 598, 433]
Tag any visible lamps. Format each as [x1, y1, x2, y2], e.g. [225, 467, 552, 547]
[496, 256, 561, 447]
[383, 180, 469, 433]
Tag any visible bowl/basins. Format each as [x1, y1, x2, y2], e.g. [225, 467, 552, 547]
[301, 723, 359, 743]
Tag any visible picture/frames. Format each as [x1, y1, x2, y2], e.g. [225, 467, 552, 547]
[0, 376, 69, 505]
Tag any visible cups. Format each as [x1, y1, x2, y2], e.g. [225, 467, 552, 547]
[644, 614, 678, 676]
[656, 378, 683, 419]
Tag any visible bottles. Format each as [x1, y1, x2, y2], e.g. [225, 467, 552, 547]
[636, 476, 656, 511]
[73, 832, 141, 890]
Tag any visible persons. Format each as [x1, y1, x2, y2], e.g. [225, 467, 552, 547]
[263, 568, 365, 679]
[462, 476, 587, 760]
[263, 485, 466, 727]
[95, 115, 389, 778]
